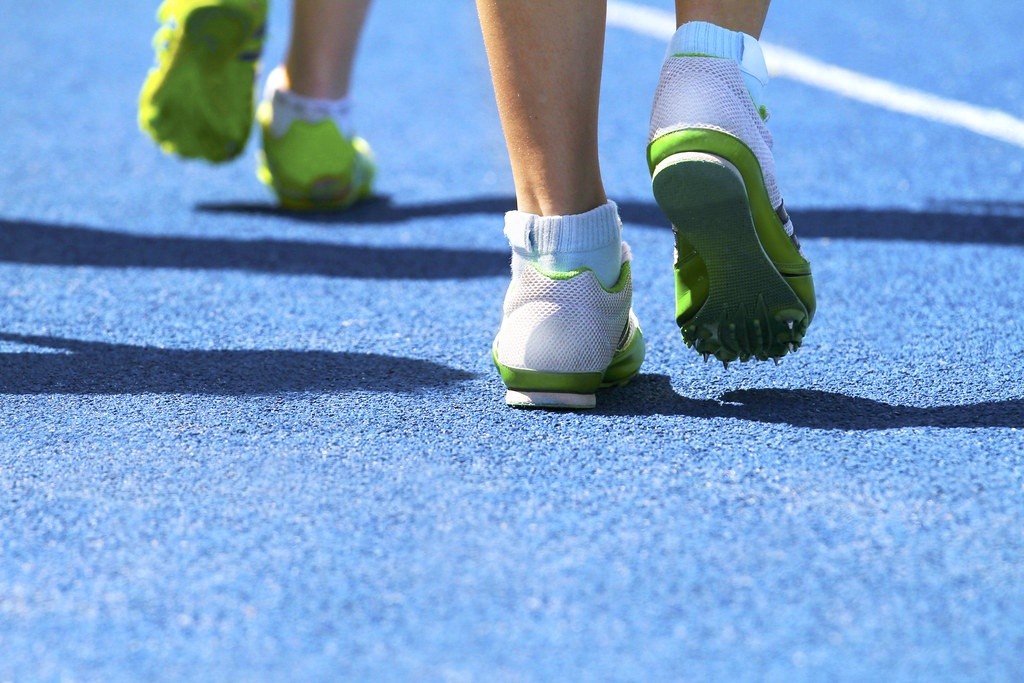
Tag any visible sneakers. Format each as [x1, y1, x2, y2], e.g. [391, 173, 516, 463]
[490, 240, 644, 407]
[136, 0, 380, 212]
[646, 52, 815, 368]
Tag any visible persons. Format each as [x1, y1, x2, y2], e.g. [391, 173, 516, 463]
[467, 1, 820, 412]
[135, 0, 382, 211]
